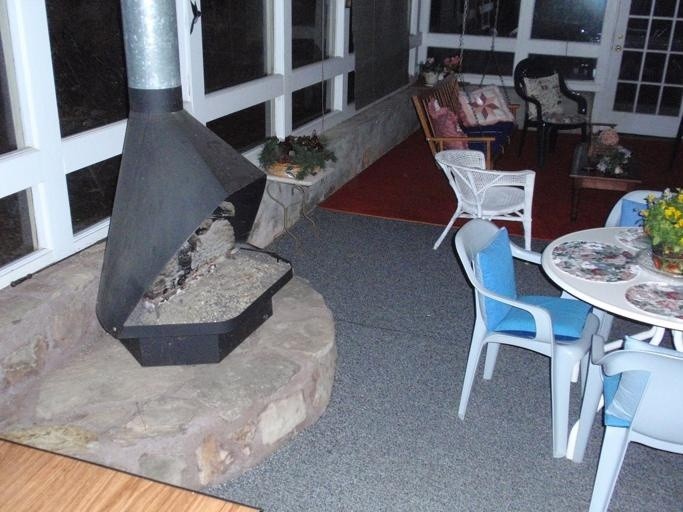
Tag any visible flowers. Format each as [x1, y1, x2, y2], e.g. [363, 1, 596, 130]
[586, 127, 632, 175]
[441, 55, 463, 73]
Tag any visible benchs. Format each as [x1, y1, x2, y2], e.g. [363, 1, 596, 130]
[411, 71, 520, 173]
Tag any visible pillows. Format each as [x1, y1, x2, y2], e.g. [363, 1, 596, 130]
[426, 87, 517, 152]
[523, 74, 564, 121]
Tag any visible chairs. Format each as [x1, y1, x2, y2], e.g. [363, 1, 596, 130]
[567, 137, 641, 222]
[604, 188, 678, 226]
[453, 219, 602, 460]
[565, 333, 683, 511]
[432, 150, 539, 267]
[512, 55, 588, 167]
[632, 187, 683, 279]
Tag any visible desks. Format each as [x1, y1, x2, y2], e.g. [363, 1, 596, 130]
[537, 225, 683, 333]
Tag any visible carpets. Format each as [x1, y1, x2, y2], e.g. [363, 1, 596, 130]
[317, 132, 681, 240]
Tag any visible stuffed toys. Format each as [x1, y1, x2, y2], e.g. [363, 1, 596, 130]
[593, 128, 633, 174]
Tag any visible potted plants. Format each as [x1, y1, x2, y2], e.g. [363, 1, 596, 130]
[423, 55, 443, 89]
[262, 135, 334, 180]
